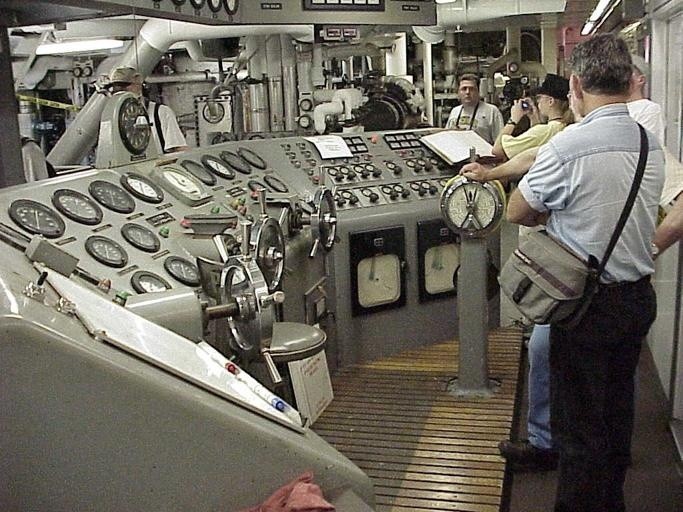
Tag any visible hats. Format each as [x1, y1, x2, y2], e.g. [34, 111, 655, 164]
[537, 74, 568, 101]
[631, 53, 648, 75]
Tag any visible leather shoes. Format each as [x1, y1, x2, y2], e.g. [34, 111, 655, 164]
[498, 438, 559, 471]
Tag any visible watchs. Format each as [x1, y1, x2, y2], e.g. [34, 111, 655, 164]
[652, 244, 659, 256]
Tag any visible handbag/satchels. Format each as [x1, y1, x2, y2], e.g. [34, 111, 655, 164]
[497, 228, 599, 328]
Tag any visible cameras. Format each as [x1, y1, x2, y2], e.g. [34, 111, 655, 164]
[522, 102, 529, 109]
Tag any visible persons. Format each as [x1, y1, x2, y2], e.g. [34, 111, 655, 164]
[21, 137, 49, 183]
[104, 67, 187, 156]
[446, 54, 665, 339]
[506, 36, 666, 512]
[461, 75, 683, 465]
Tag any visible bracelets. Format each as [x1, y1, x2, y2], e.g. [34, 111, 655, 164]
[508, 119, 517, 126]
